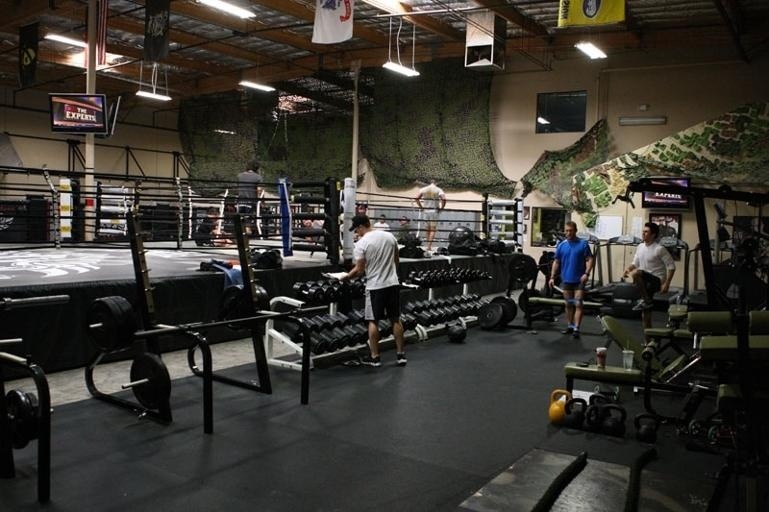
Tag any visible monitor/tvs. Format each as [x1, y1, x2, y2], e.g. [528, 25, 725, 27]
[47, 93, 110, 135]
[107, 96, 122, 136]
[536, 89, 588, 134]
[642, 177, 694, 212]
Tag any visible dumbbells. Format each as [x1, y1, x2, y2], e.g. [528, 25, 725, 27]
[293, 268, 492, 300]
[273, 293, 488, 355]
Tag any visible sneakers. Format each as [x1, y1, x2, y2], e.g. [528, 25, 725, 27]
[396, 352, 407, 363]
[632, 300, 653, 311]
[562, 328, 579, 337]
[362, 354, 381, 366]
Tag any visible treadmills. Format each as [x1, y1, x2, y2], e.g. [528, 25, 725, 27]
[584, 226, 733, 313]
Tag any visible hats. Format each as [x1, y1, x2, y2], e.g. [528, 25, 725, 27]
[349, 215, 369, 231]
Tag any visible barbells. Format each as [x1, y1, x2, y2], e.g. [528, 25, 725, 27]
[509, 252, 556, 282]
[88, 283, 271, 349]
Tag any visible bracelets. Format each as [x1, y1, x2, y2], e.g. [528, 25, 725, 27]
[584, 273, 590, 276]
[550, 277, 554, 279]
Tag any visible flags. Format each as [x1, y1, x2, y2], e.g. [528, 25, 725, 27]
[144, 0, 169, 63]
[311, 0, 354, 44]
[18, 19, 40, 86]
[557, 0, 627, 30]
[81, 0, 107, 65]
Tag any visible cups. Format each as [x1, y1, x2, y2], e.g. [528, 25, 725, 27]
[595, 346, 607, 371]
[623, 350, 635, 371]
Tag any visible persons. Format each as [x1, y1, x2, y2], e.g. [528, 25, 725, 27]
[548, 222, 593, 336]
[338, 215, 409, 366]
[623, 222, 676, 348]
[237, 159, 266, 238]
[416, 179, 446, 250]
[195, 197, 414, 248]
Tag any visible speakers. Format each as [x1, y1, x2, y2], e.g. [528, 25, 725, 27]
[464, 12, 508, 73]
